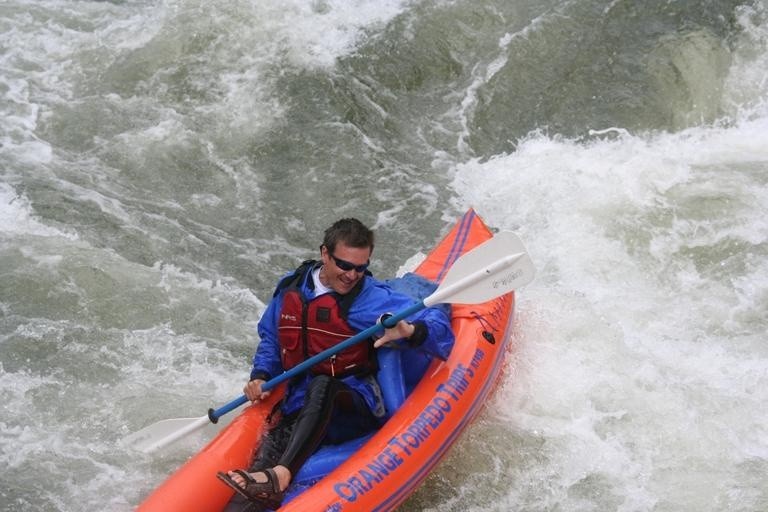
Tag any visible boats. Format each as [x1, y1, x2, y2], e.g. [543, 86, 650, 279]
[131, 202, 517, 512]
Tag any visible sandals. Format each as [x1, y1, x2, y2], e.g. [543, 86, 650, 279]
[215, 469, 288, 511]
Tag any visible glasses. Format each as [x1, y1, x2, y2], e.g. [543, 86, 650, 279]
[329, 253, 369, 273]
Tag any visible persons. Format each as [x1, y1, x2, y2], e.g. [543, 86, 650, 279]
[215, 216, 458, 512]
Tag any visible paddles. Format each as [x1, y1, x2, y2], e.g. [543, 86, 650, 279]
[121, 231, 535, 454]
